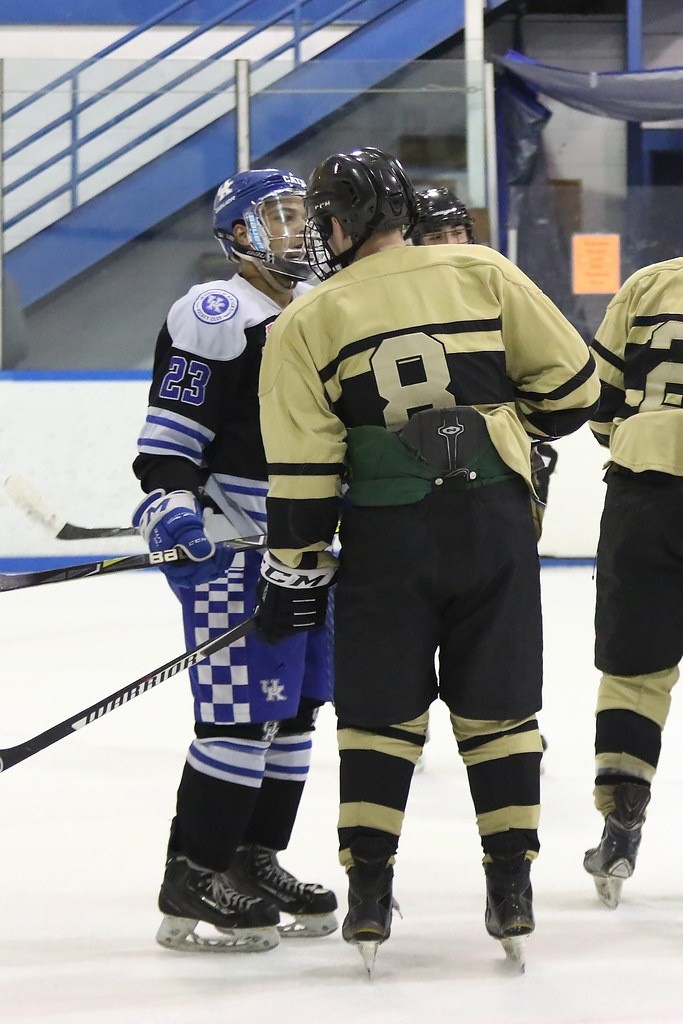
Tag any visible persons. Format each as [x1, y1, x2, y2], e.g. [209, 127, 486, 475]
[251, 148, 601, 979]
[406, 187, 476, 246]
[583, 256, 683, 907]
[131, 170, 339, 952]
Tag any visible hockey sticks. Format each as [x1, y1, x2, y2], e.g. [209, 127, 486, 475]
[0, 468, 142, 541]
[0, 532, 269, 596]
[0, 612, 259, 775]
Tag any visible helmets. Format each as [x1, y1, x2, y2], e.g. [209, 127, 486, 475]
[301, 146, 418, 267]
[411, 187, 476, 244]
[212, 168, 329, 282]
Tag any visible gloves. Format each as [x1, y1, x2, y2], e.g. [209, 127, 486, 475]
[251, 551, 340, 646]
[132, 487, 236, 587]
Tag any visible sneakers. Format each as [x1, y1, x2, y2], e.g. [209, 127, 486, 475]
[483, 860, 535, 973]
[583, 783, 651, 910]
[342, 865, 403, 979]
[220, 844, 339, 938]
[155, 855, 281, 952]
